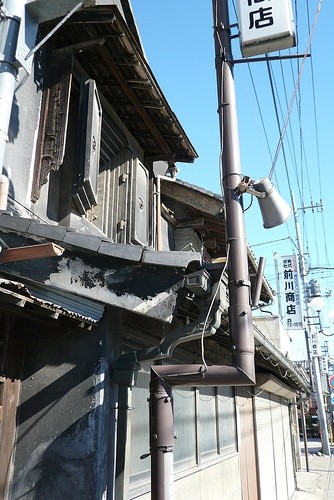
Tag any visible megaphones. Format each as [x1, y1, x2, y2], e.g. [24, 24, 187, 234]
[239, 178, 292, 228]
